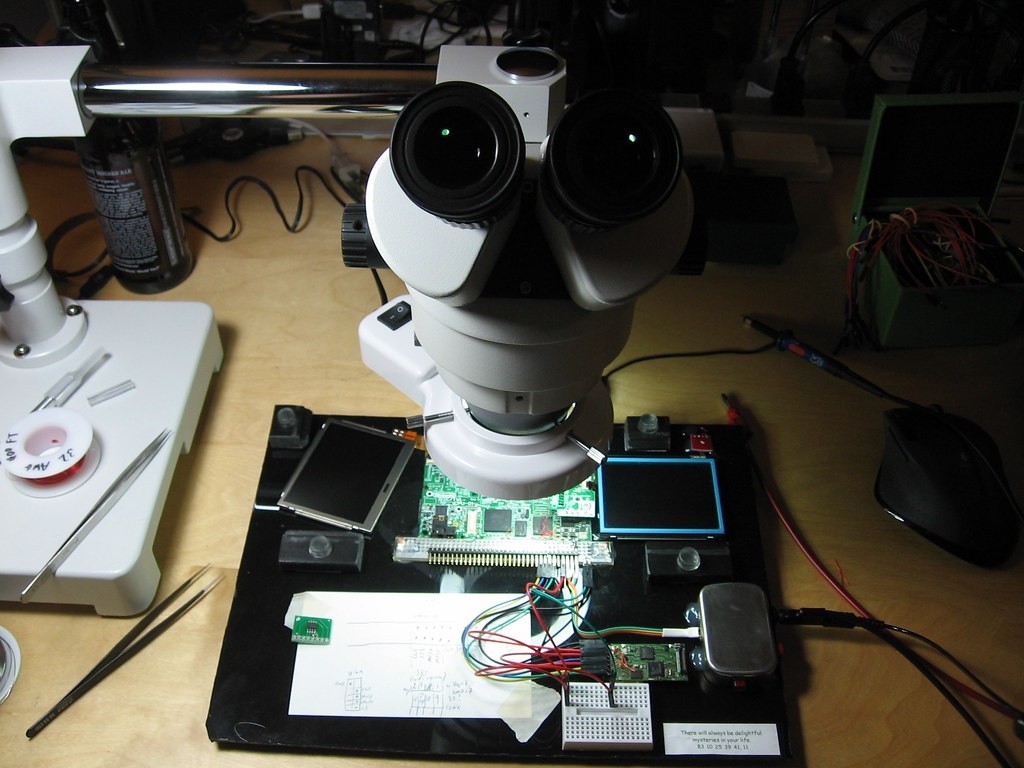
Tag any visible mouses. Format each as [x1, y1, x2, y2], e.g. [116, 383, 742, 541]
[873, 405, 1020, 571]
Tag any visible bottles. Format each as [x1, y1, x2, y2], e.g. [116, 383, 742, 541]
[48, 0, 195, 295]
